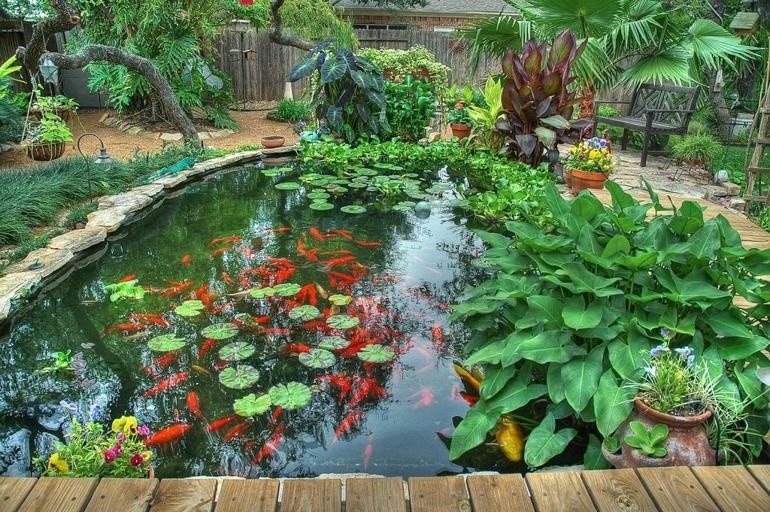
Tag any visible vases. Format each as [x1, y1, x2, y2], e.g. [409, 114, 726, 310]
[448, 122, 472, 140]
[561, 165, 580, 188]
[571, 168, 610, 195]
[601, 390, 732, 467]
[554, 159, 567, 177]
[144, 462, 156, 480]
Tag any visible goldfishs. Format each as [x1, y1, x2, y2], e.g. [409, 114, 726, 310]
[103, 226, 480, 477]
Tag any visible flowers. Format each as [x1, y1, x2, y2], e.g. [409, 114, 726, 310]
[560, 136, 618, 175]
[31, 415, 154, 477]
[614, 327, 747, 414]
[445, 103, 479, 129]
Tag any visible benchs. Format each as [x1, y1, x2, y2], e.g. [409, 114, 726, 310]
[590, 80, 701, 167]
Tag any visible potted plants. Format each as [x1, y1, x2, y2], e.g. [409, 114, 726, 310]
[21, 111, 75, 162]
[28, 83, 81, 124]
